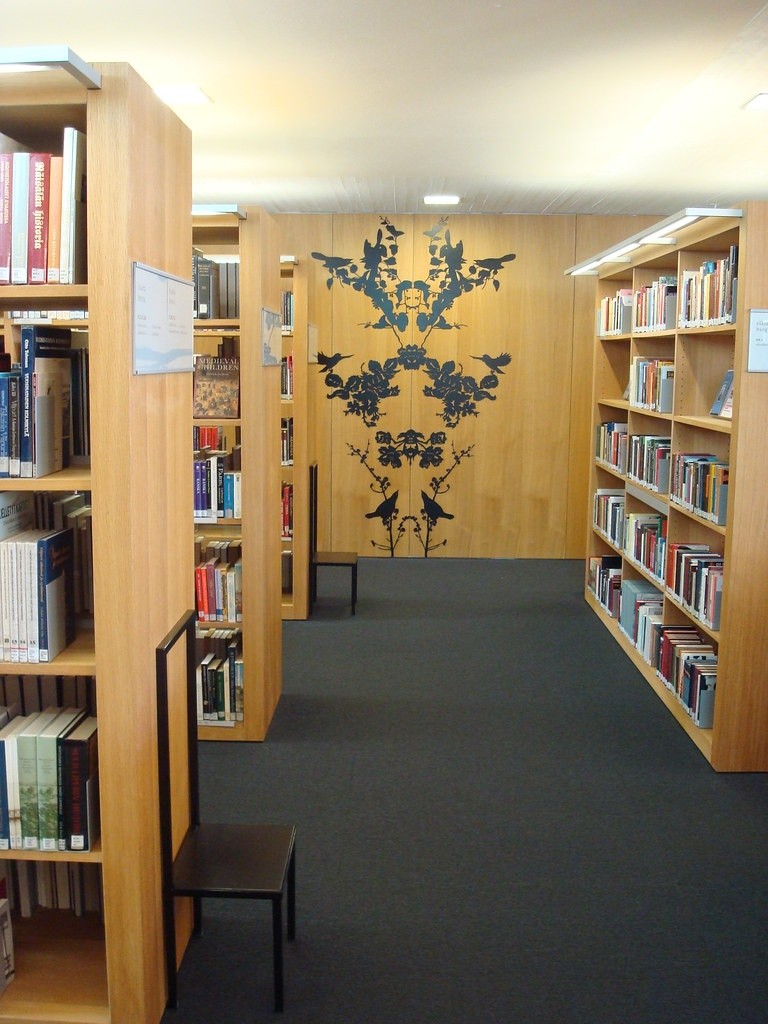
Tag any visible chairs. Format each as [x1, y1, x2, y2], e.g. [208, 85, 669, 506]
[309, 460, 358, 615]
[155, 609, 298, 1014]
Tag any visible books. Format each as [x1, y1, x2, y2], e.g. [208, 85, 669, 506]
[279, 290, 294, 537]
[0, 128, 105, 990]
[593, 488, 724, 631]
[192, 244, 246, 724]
[595, 421, 729, 526]
[622, 356, 735, 419]
[587, 555, 718, 729]
[600, 246, 738, 336]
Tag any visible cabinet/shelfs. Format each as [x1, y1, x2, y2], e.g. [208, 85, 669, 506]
[279, 254, 318, 620]
[586, 201, 768, 772]
[192, 205, 283, 740]
[0, 63, 195, 1022]
